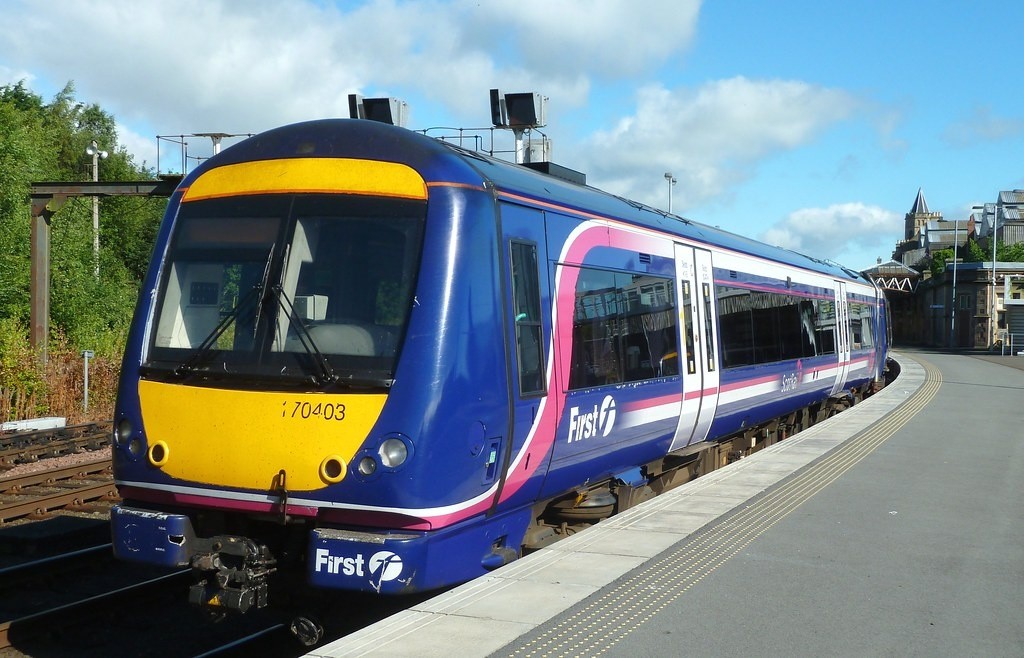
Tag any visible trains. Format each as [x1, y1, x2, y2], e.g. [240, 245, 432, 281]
[111, 117, 894, 619]
